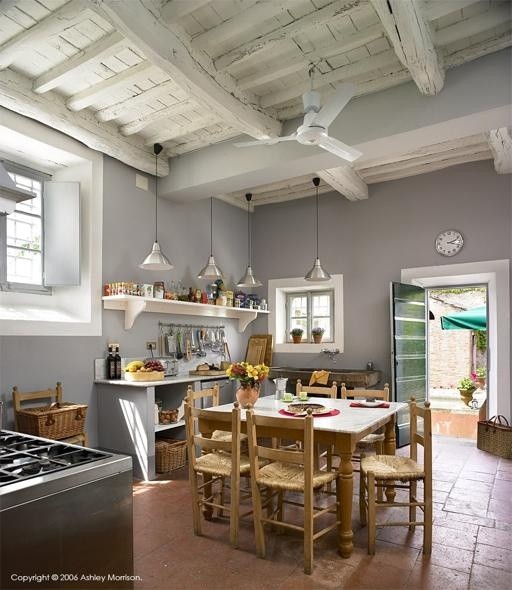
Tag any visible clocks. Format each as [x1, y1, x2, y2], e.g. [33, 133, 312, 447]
[435, 229, 463, 257]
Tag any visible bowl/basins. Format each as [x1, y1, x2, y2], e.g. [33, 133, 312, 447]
[125, 371, 165, 382]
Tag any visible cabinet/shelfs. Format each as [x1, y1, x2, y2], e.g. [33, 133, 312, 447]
[97, 381, 195, 481]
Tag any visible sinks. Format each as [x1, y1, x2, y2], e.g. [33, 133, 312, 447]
[268, 367, 381, 388]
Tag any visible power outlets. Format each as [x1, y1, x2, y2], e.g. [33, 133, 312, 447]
[146, 342, 157, 351]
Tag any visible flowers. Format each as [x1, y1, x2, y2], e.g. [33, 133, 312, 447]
[225, 361, 270, 384]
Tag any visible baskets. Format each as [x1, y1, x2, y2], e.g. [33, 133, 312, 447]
[155, 439, 187, 473]
[16, 406, 86, 440]
[477, 415, 512, 458]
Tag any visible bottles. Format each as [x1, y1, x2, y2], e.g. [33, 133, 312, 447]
[115, 346, 122, 379]
[153, 280, 218, 305]
[106, 347, 115, 379]
[261, 298, 268, 310]
[367, 359, 373, 372]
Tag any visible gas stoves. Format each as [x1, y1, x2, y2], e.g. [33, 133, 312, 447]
[0, 428, 133, 511]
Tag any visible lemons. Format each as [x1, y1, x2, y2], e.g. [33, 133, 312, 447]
[129, 366, 137, 372]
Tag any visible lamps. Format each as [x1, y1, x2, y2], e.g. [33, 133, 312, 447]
[138, 141, 173, 272]
[306, 177, 332, 282]
[197, 195, 223, 280]
[236, 191, 264, 289]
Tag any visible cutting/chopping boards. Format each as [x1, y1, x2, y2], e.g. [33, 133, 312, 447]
[246, 345, 263, 365]
[249, 335, 272, 366]
[189, 369, 226, 376]
[245, 338, 267, 365]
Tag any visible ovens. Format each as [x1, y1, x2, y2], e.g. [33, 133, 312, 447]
[201, 377, 238, 408]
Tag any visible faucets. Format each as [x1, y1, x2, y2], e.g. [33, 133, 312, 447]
[325, 350, 337, 364]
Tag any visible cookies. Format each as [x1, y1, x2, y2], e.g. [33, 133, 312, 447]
[159, 412, 178, 424]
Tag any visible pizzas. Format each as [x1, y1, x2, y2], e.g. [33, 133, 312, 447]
[287, 403, 324, 412]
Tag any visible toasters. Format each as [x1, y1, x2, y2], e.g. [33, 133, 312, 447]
[145, 356, 181, 377]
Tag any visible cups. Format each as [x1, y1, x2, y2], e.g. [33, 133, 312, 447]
[283, 393, 292, 400]
[274, 378, 286, 400]
[298, 392, 307, 400]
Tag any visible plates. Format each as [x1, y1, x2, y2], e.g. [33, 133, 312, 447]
[284, 405, 331, 416]
[298, 397, 310, 401]
[280, 398, 296, 403]
[356, 400, 386, 407]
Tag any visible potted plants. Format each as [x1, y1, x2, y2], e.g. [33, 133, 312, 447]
[457, 377, 476, 402]
[476, 368, 487, 389]
[311, 327, 326, 343]
[289, 328, 304, 344]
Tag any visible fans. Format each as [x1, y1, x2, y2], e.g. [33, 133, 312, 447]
[234, 58, 363, 164]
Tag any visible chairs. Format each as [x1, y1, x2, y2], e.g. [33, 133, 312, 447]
[13, 382, 89, 447]
[183, 378, 433, 576]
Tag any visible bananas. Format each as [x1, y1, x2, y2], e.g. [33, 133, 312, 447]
[126, 361, 145, 370]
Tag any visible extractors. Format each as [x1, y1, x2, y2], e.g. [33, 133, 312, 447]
[0, 162, 37, 217]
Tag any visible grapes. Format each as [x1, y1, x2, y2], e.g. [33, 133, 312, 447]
[146, 360, 164, 371]
